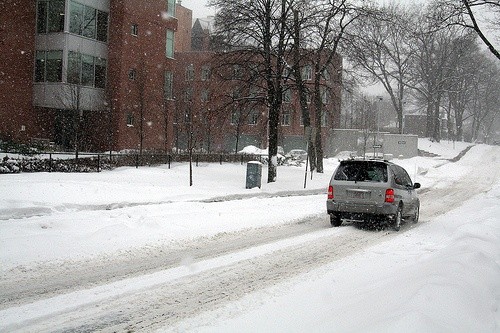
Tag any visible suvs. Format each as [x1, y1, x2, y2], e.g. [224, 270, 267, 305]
[326, 158, 420, 231]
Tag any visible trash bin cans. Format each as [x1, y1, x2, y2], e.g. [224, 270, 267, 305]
[246, 161, 262, 189]
